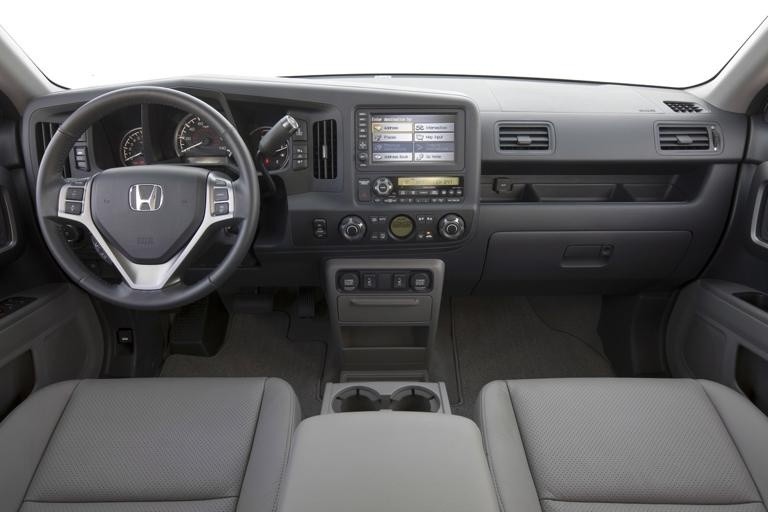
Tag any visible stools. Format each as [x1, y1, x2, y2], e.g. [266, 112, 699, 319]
[0, 359, 298, 511]
[472, 374, 768, 512]
